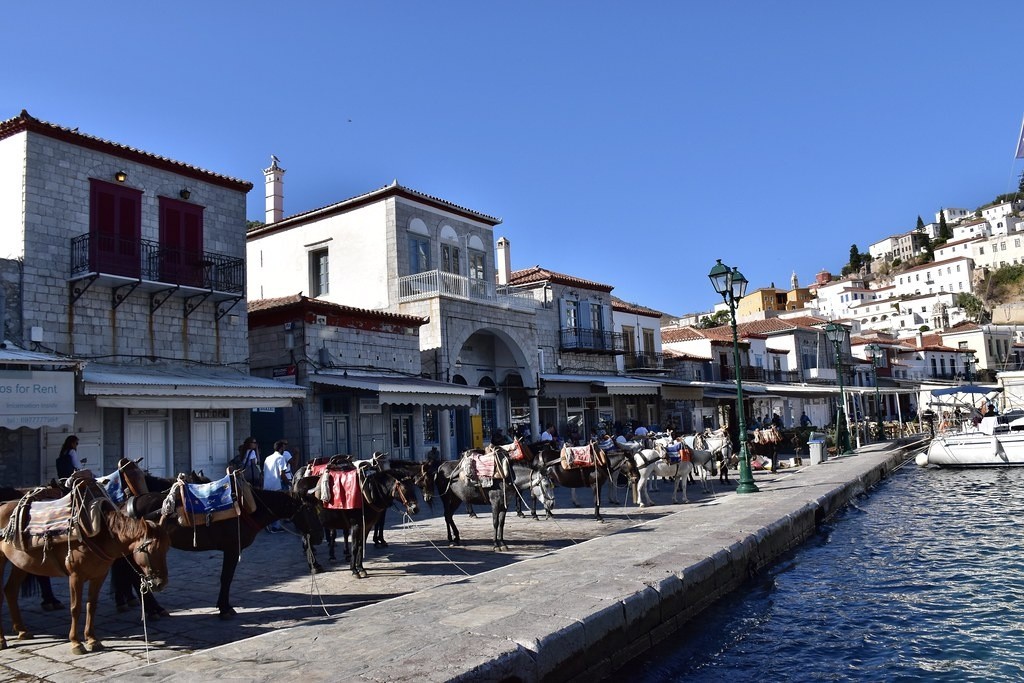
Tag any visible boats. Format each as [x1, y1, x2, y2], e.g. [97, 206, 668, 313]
[913, 384, 1024, 469]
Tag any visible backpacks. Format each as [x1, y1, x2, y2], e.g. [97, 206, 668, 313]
[56, 448, 78, 481]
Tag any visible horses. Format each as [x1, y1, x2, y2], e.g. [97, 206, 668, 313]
[288, 457, 433, 581]
[433, 426, 734, 552]
[732, 429, 802, 474]
[0, 469, 325, 655]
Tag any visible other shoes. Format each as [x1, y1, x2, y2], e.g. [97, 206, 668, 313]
[278, 527, 286, 532]
[271, 527, 277, 533]
[265, 525, 271, 533]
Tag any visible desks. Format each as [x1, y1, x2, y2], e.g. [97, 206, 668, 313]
[191, 260, 215, 289]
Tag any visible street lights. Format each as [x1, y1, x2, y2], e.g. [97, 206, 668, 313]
[863, 342, 887, 442]
[706, 257, 760, 494]
[825, 321, 855, 456]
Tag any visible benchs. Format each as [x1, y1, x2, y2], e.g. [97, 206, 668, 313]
[410, 281, 442, 295]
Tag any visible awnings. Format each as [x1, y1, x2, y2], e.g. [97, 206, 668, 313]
[636, 376, 766, 400]
[591, 380, 662, 395]
[1, 349, 78, 430]
[82, 371, 307, 408]
[312, 373, 485, 406]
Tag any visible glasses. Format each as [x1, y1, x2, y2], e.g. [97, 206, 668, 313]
[283, 443, 288, 445]
[76, 443, 79, 445]
[251, 442, 257, 443]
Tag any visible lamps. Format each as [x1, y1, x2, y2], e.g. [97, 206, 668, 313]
[180, 190, 191, 200]
[115, 170, 127, 183]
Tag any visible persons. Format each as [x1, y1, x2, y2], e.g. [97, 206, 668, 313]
[984, 404, 999, 417]
[857, 408, 863, 419]
[909, 404, 914, 412]
[590, 424, 648, 441]
[490, 427, 503, 447]
[509, 428, 514, 439]
[58, 436, 86, 470]
[428, 447, 439, 461]
[746, 413, 784, 430]
[230, 436, 298, 533]
[955, 407, 960, 417]
[944, 411, 950, 418]
[850, 415, 855, 422]
[800, 411, 812, 427]
[542, 424, 562, 449]
[524, 425, 531, 435]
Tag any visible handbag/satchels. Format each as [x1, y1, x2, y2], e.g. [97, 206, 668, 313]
[242, 464, 259, 480]
